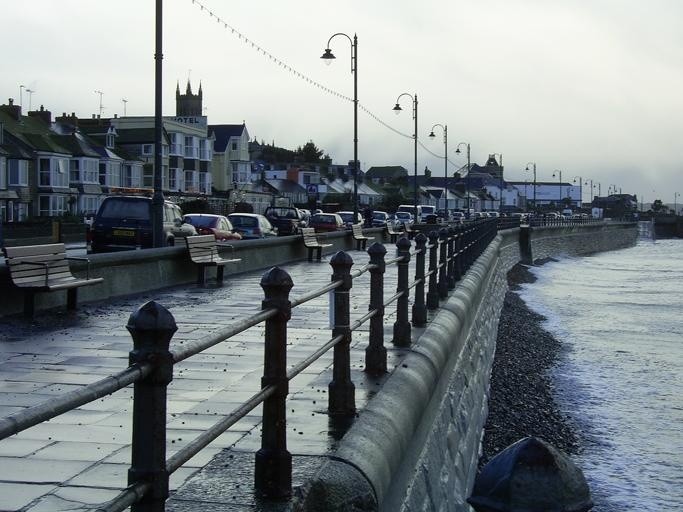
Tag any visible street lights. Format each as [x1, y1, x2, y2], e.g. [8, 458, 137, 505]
[573, 175, 621, 219]
[455, 143, 470, 220]
[525, 162, 536, 216]
[428, 124, 448, 222]
[392, 93, 418, 225]
[675, 191, 681, 216]
[319, 31, 358, 226]
[552, 169, 562, 218]
[488, 152, 503, 217]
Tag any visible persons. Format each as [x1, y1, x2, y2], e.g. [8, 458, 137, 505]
[301, 211, 309, 228]
[364, 204, 373, 228]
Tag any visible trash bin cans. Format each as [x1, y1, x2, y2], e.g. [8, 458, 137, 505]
[277, 218, 300, 237]
[426, 214, 438, 224]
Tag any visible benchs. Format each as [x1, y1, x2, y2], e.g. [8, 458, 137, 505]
[402, 221, 419, 240]
[0, 238, 106, 322]
[385, 219, 404, 244]
[350, 223, 376, 252]
[299, 226, 334, 264]
[182, 233, 243, 285]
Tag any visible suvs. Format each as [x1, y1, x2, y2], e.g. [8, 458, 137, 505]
[86, 186, 199, 255]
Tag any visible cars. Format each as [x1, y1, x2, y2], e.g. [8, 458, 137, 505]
[183, 213, 243, 243]
[226, 213, 279, 240]
